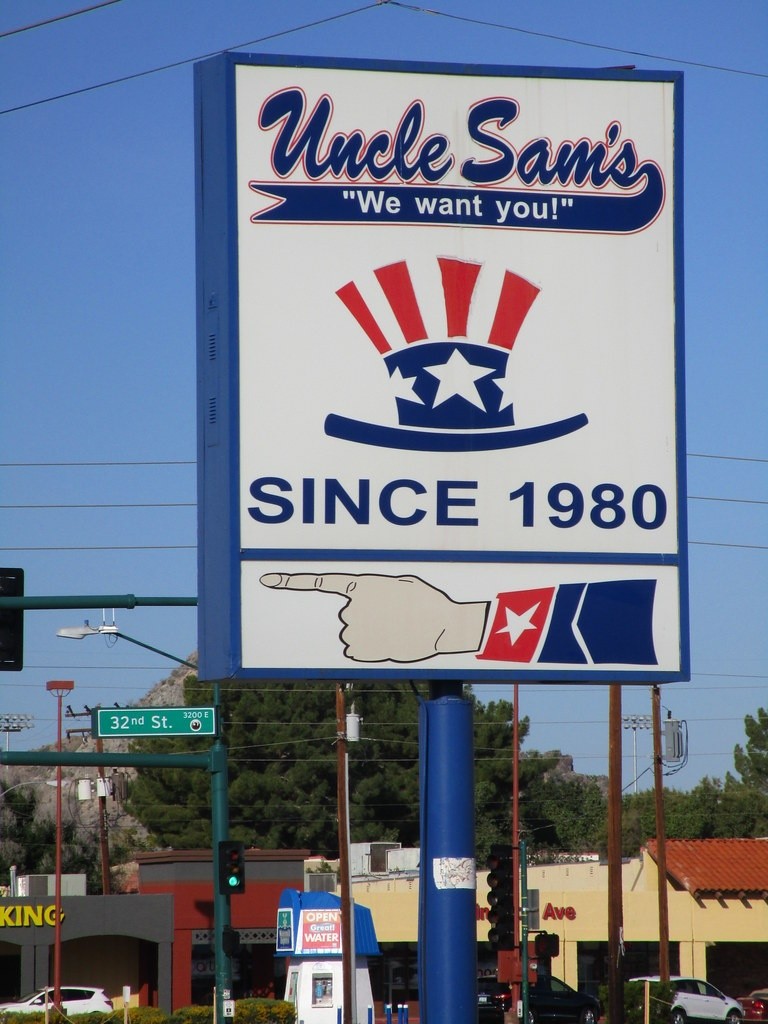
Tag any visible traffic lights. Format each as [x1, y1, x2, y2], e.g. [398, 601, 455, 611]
[486, 852, 517, 949]
[219, 840, 246, 894]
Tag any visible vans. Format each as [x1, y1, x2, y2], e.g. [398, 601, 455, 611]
[477, 974, 601, 1024]
[629, 977, 744, 1024]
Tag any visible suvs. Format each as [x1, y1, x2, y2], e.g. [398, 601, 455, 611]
[0, 987, 113, 1017]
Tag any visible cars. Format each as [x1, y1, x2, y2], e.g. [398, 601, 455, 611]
[737, 989, 768, 1024]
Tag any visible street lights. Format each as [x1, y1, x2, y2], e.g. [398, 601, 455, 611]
[58, 627, 236, 1024]
